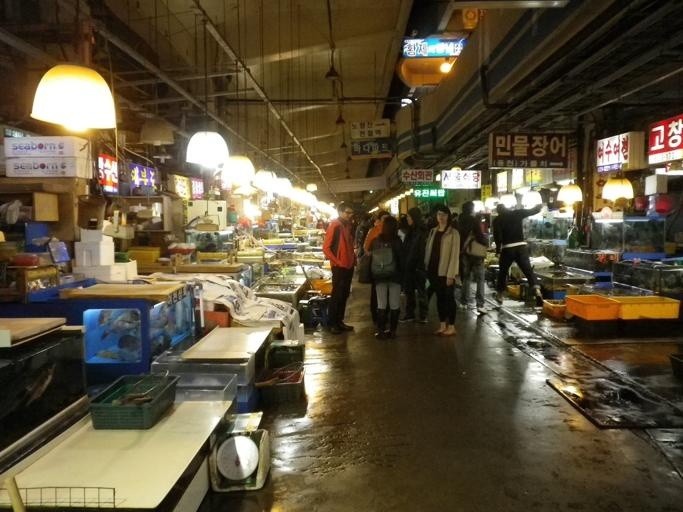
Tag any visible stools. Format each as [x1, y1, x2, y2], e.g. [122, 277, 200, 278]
[519, 277, 542, 304]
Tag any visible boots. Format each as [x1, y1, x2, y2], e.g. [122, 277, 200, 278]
[384, 307, 401, 338]
[374, 309, 386, 340]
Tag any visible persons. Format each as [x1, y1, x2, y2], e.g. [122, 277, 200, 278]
[450, 212, 458, 229]
[317, 219, 329, 232]
[458, 202, 474, 289]
[459, 202, 490, 314]
[367, 216, 407, 339]
[492, 202, 547, 307]
[363, 211, 390, 326]
[423, 204, 461, 336]
[398, 213, 409, 244]
[352, 218, 357, 244]
[322, 203, 357, 335]
[423, 212, 439, 247]
[398, 207, 429, 323]
[357, 205, 380, 258]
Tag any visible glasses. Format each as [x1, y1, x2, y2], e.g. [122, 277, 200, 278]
[345, 212, 354, 216]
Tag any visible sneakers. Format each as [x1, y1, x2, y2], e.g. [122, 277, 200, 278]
[337, 321, 353, 331]
[440, 329, 457, 337]
[328, 326, 343, 334]
[473, 306, 488, 314]
[535, 288, 544, 306]
[459, 304, 468, 310]
[492, 293, 502, 305]
[418, 316, 429, 323]
[433, 329, 446, 336]
[399, 316, 415, 322]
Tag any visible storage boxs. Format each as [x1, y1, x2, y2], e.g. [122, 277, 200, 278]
[73, 228, 137, 284]
[539, 298, 568, 318]
[234, 379, 255, 402]
[506, 283, 522, 299]
[4, 155, 95, 180]
[606, 295, 681, 321]
[150, 350, 255, 387]
[156, 372, 239, 400]
[665, 351, 682, 380]
[259, 371, 304, 402]
[564, 293, 621, 320]
[0, 131, 91, 159]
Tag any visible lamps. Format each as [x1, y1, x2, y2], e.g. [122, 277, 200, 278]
[28, 61, 118, 135]
[138, 116, 176, 147]
[496, 99, 634, 208]
[180, 0, 342, 221]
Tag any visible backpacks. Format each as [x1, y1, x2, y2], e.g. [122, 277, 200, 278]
[371, 243, 397, 280]
[357, 227, 369, 244]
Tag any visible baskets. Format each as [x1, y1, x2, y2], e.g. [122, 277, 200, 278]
[100, 382, 167, 406]
[88, 375, 181, 430]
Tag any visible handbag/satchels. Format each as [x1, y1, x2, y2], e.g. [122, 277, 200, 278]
[462, 229, 488, 259]
[359, 256, 371, 284]
